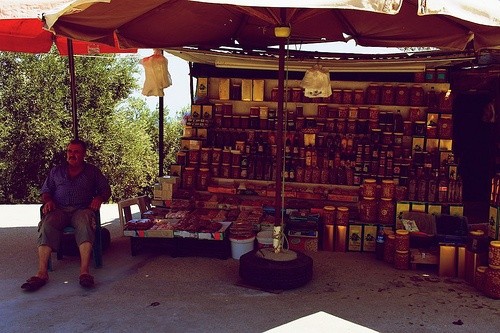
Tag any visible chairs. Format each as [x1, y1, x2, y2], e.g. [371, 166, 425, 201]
[39, 201, 103, 269]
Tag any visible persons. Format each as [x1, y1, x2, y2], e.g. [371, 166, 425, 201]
[20, 139, 112, 291]
[454, 97, 500, 224]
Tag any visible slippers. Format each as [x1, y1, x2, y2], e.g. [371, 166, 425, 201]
[79, 274, 94, 286]
[21, 275, 49, 289]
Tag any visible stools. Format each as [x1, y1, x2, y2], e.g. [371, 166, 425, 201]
[117, 194, 152, 231]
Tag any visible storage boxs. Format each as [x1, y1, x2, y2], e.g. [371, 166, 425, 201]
[286, 229, 319, 257]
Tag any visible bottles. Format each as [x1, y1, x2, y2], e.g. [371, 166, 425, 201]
[290, 161, 296, 182]
[293, 137, 299, 157]
[429, 87, 435, 107]
[285, 160, 290, 182]
[286, 136, 291, 156]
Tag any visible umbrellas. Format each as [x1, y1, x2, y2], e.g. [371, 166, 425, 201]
[0, 1, 137, 140]
[38, 1, 500, 292]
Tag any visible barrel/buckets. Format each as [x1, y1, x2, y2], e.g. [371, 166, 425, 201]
[229, 236, 256, 259]
[256, 231, 284, 250]
